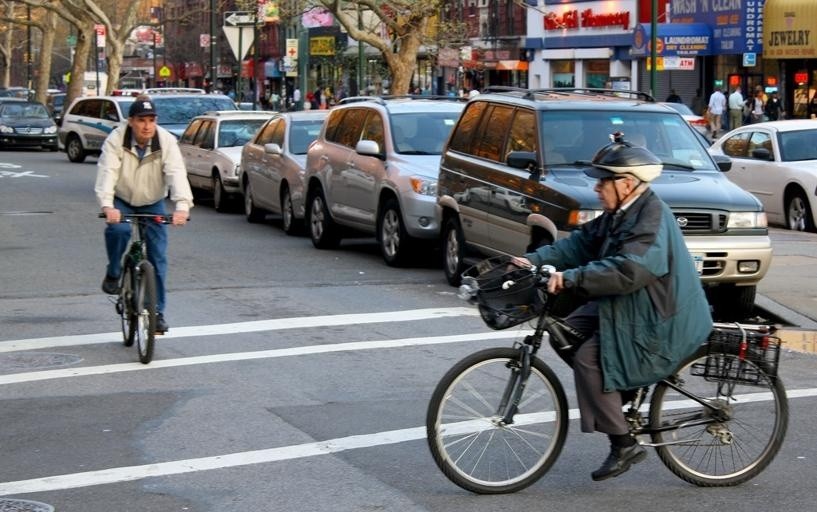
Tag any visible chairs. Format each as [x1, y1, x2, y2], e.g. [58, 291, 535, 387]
[623, 131, 647, 149]
[543, 134, 568, 165]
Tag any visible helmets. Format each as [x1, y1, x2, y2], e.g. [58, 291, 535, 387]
[581, 140, 664, 182]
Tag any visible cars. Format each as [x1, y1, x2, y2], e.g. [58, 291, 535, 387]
[235, 108, 332, 236]
[173, 109, 280, 215]
[0, 79, 279, 151]
[703, 116, 817, 233]
[659, 99, 709, 135]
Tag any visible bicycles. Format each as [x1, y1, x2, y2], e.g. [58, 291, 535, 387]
[420, 252, 794, 499]
[95, 208, 193, 367]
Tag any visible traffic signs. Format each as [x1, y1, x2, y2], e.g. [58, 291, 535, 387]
[221, 9, 266, 27]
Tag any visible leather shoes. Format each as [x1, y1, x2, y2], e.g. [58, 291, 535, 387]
[102, 276, 120, 294]
[591, 438, 647, 482]
[156, 314, 168, 332]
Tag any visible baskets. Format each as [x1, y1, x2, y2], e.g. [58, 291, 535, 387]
[459, 253, 546, 329]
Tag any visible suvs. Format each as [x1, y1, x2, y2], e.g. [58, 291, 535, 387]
[303, 92, 476, 267]
[429, 80, 776, 319]
[57, 93, 137, 164]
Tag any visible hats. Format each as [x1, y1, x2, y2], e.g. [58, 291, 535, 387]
[129, 100, 158, 118]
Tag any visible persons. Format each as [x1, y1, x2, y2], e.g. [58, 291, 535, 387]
[806, 92, 817, 120]
[509, 142, 714, 481]
[94, 101, 194, 331]
[269, 81, 348, 112]
[665, 85, 787, 138]
[408, 82, 480, 101]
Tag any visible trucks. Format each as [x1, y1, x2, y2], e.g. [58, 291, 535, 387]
[62, 69, 109, 97]
